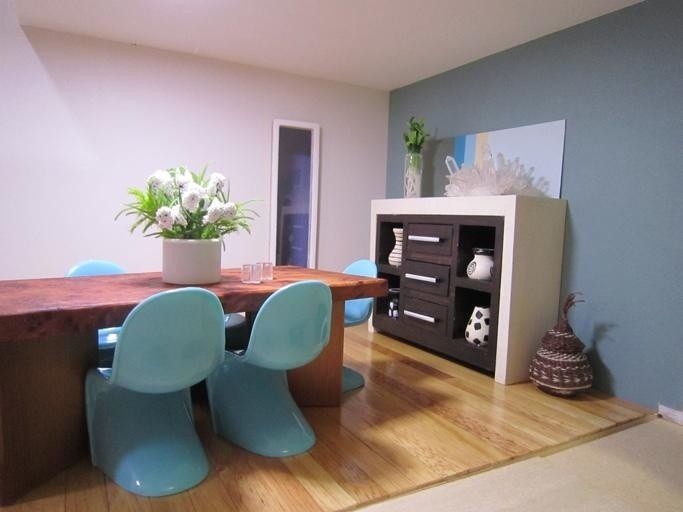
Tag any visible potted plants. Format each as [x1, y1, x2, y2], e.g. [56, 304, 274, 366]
[401, 113, 430, 198]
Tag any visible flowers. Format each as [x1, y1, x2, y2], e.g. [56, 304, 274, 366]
[114, 160, 265, 251]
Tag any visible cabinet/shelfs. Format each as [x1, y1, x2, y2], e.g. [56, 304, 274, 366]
[369, 214, 505, 378]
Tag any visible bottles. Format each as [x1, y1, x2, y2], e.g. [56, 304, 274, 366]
[388, 286, 401, 317]
[389, 228, 403, 267]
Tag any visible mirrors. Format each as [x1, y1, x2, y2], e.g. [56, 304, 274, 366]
[268, 118, 320, 269]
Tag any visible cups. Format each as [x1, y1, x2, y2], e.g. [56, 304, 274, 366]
[241, 263, 273, 284]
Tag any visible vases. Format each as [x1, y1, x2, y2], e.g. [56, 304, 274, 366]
[388, 227, 404, 266]
[162, 237, 222, 286]
[387, 288, 401, 319]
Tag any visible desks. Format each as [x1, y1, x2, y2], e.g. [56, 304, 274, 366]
[0, 265, 388, 508]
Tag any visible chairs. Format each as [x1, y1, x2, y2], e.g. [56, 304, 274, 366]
[84, 286, 226, 496]
[69, 260, 128, 276]
[203, 281, 332, 459]
[338, 259, 377, 394]
